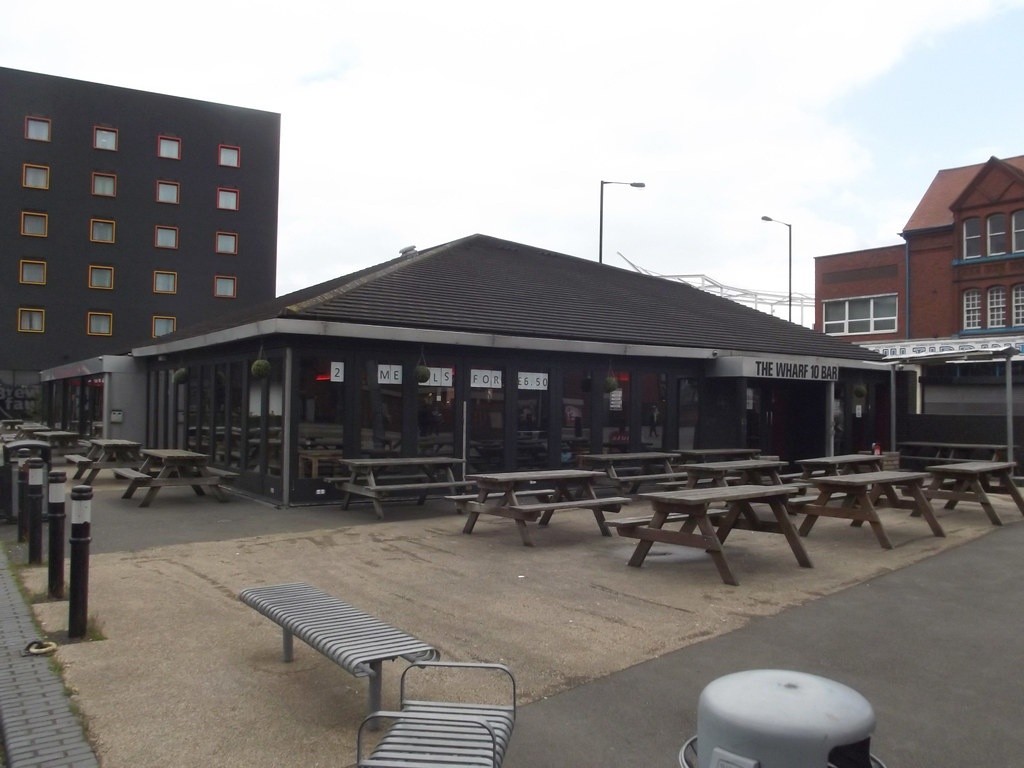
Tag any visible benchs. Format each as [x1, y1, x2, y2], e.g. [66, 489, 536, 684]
[0, 431, 230, 509]
[356, 660, 516, 768]
[324, 455, 961, 571]
[238, 582, 442, 731]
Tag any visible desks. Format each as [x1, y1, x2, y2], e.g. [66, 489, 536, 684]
[337, 446, 1024, 587]
[1, 419, 230, 508]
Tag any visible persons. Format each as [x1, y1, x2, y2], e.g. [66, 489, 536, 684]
[649, 406, 660, 438]
